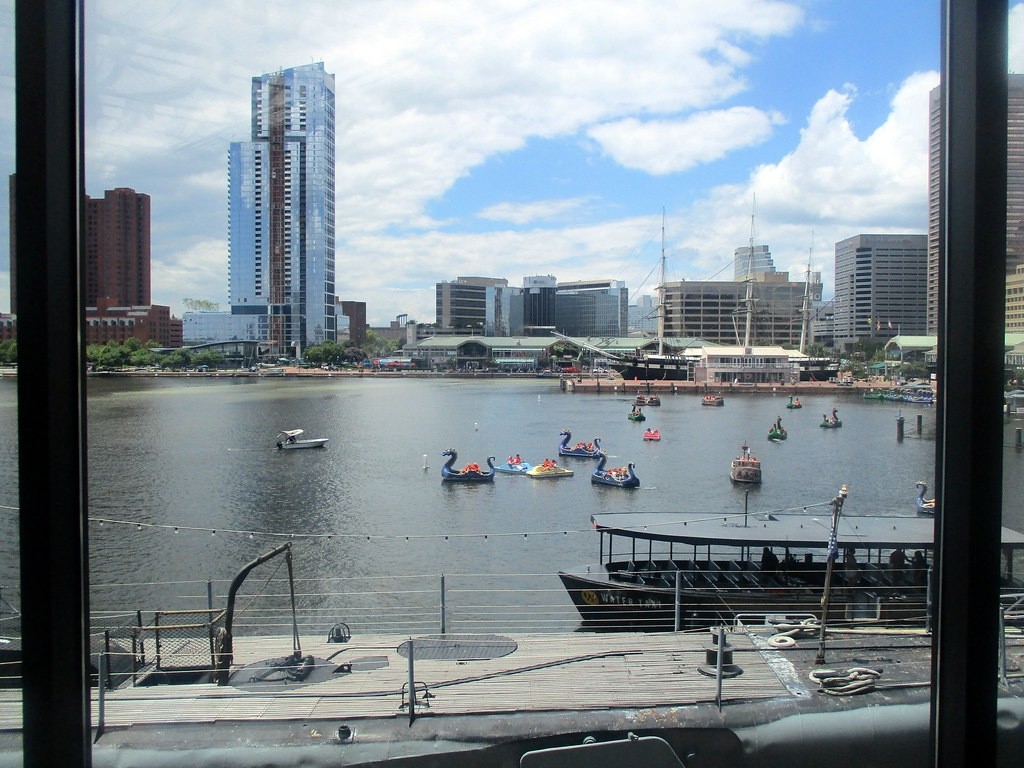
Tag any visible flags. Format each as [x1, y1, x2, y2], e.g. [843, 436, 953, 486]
[868, 318, 872, 324]
[877, 321, 880, 330]
[888, 321, 892, 329]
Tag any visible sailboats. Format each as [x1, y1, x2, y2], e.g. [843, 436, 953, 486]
[537, 190, 840, 382]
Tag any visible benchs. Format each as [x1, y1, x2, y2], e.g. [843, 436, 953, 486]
[619, 559, 928, 592]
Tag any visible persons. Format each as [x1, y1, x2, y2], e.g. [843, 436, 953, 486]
[544, 458, 557, 469]
[890, 549, 907, 580]
[508, 454, 522, 464]
[759, 548, 778, 585]
[909, 550, 926, 585]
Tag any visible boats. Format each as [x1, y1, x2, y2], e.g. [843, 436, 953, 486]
[627, 402, 647, 421]
[635, 396, 649, 408]
[590, 449, 642, 488]
[914, 481, 936, 515]
[862, 381, 935, 404]
[526, 464, 574, 479]
[438, 448, 496, 482]
[700, 393, 725, 407]
[557, 488, 1024, 633]
[558, 428, 605, 458]
[646, 393, 661, 406]
[818, 407, 843, 428]
[728, 440, 762, 484]
[767, 415, 788, 441]
[785, 394, 803, 409]
[493, 460, 535, 475]
[643, 430, 662, 441]
[273, 429, 330, 450]
